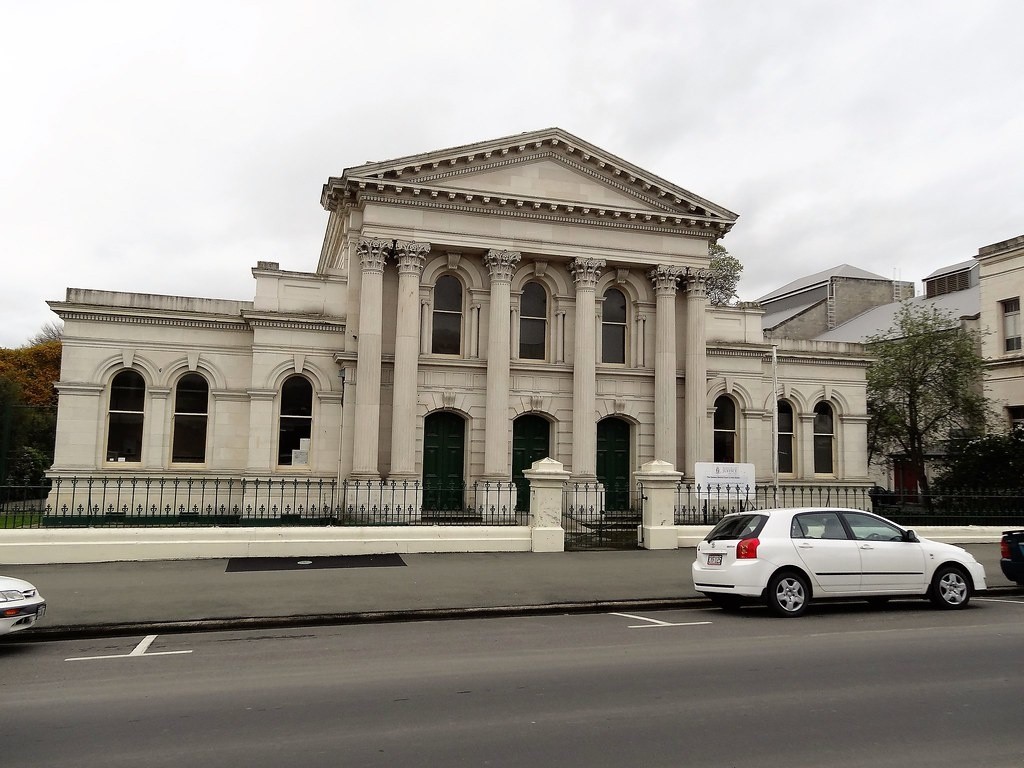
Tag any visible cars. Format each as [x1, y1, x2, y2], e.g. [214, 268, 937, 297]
[0, 575, 47, 640]
[691, 507, 988, 616]
[1000, 528, 1024, 589]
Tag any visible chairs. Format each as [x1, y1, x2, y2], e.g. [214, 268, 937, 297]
[821, 518, 846, 539]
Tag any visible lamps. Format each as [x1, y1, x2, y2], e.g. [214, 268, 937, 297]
[794, 522, 808, 536]
